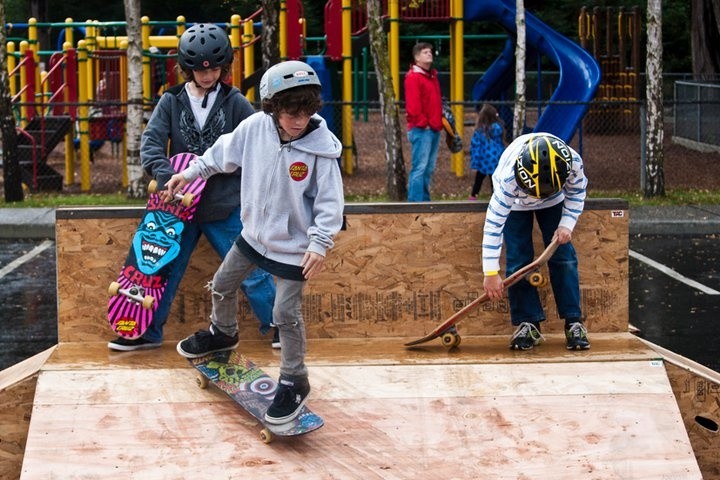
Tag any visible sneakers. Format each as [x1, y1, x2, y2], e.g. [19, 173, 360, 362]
[107, 335, 164, 351]
[508, 322, 542, 351]
[175, 322, 240, 359]
[271, 326, 282, 349]
[263, 374, 312, 425]
[563, 317, 591, 351]
[466, 195, 477, 200]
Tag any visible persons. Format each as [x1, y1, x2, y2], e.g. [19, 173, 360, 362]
[469, 104, 504, 201]
[482, 131, 590, 350]
[109, 24, 283, 351]
[163, 61, 345, 425]
[404, 43, 444, 202]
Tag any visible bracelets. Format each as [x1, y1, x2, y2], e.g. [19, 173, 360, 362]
[484, 272, 498, 276]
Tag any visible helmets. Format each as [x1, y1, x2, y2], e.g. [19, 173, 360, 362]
[259, 60, 323, 103]
[178, 22, 234, 71]
[514, 135, 572, 200]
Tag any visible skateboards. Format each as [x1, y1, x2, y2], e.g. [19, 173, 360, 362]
[403, 234, 560, 349]
[106, 152, 208, 341]
[185, 348, 325, 445]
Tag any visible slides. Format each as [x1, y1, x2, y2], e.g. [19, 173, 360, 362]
[461, 0, 601, 146]
